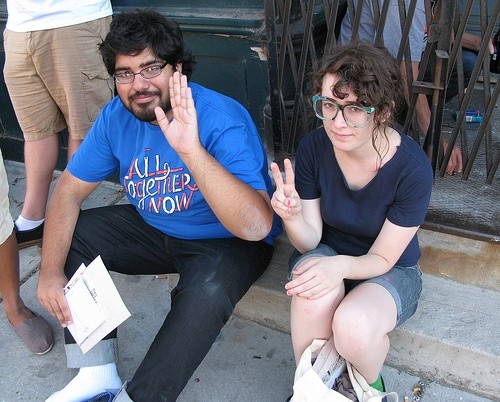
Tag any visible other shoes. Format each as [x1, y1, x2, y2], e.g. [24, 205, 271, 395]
[14, 219, 48, 250]
[5, 304, 54, 355]
[379, 374, 387, 402]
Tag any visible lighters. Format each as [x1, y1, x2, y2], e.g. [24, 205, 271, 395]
[464, 110, 480, 116]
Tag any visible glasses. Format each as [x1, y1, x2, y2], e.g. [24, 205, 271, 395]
[312, 92, 385, 129]
[113, 62, 168, 83]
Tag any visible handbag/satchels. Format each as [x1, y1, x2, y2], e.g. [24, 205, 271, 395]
[287, 336, 400, 402]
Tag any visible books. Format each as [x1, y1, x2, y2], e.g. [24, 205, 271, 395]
[312, 336, 346, 391]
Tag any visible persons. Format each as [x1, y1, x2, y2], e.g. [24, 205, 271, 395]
[3, 0, 114, 251]
[36, 8, 283, 402]
[0, 150, 55, 356]
[271, 40, 433, 402]
[339, 0, 495, 176]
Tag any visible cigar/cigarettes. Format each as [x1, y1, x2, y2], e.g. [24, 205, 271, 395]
[155, 275, 166, 279]
[448, 172, 456, 176]
